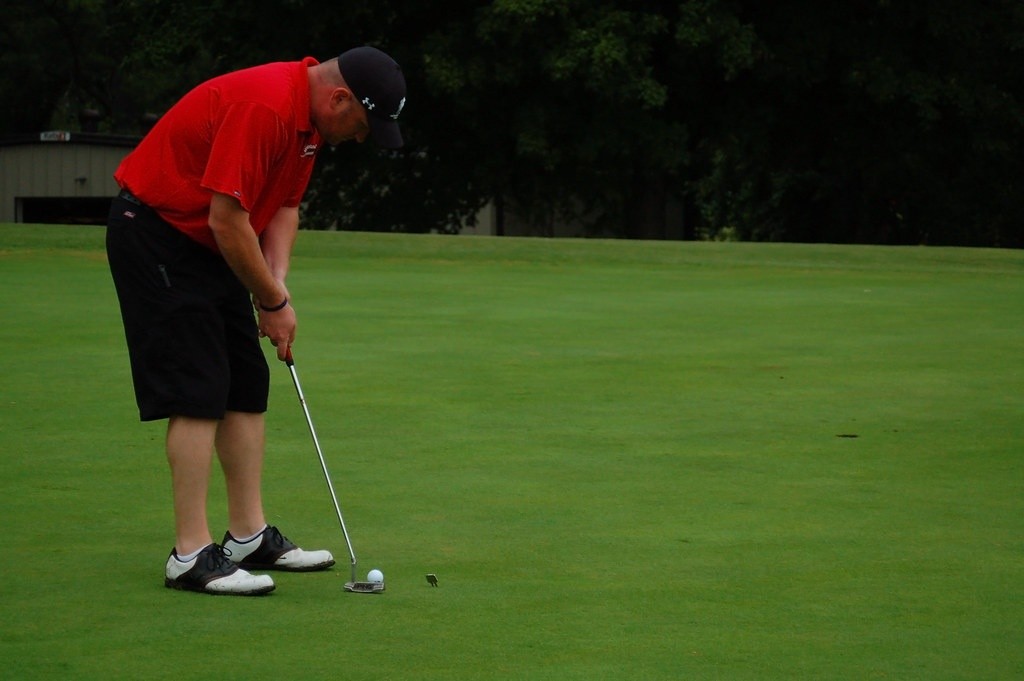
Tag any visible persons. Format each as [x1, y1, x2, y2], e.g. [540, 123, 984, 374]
[104, 45, 407, 602]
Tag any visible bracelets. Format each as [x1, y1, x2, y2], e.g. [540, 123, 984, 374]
[259, 295, 288, 312]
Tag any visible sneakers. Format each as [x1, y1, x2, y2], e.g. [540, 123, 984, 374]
[221, 527, 336, 571]
[164, 545, 276, 597]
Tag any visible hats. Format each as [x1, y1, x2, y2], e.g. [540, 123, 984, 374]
[339, 45, 407, 149]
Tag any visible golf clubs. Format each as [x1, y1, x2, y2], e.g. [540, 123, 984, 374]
[282, 343, 384, 593]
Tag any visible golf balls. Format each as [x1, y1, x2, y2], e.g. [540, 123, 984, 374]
[368, 567, 382, 585]
